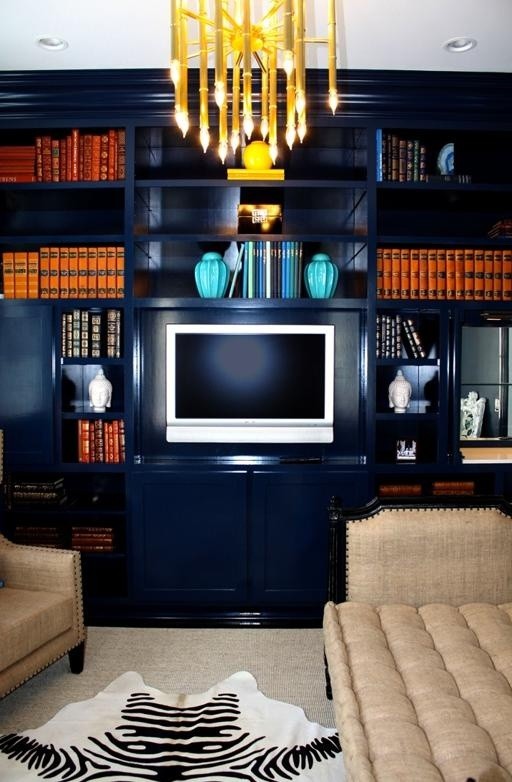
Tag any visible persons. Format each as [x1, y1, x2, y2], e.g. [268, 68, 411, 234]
[387, 369, 412, 413]
[87, 368, 113, 412]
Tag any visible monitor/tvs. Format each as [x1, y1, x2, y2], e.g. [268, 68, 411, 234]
[164, 324, 336, 443]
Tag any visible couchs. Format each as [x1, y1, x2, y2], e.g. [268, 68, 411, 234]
[322, 495, 512, 782]
[0, 532, 86, 711]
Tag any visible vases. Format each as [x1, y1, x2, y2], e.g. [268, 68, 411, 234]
[303, 251, 341, 300]
[190, 250, 231, 300]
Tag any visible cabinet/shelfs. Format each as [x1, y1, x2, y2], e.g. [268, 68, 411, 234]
[369, 309, 512, 498]
[130, 466, 371, 613]
[0, 303, 129, 617]
[0, 112, 509, 320]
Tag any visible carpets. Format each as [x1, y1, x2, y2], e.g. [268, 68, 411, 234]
[1, 671, 343, 782]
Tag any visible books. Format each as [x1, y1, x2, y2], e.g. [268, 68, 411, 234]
[460, 445, 512, 465]
[75, 419, 127, 465]
[431, 489, 476, 497]
[2, 124, 128, 357]
[5, 475, 118, 555]
[373, 308, 446, 363]
[375, 128, 435, 184]
[374, 245, 512, 301]
[226, 240, 305, 300]
[378, 483, 424, 497]
[432, 480, 476, 491]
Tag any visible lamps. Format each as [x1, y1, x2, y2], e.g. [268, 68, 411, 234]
[166, 2, 338, 167]
